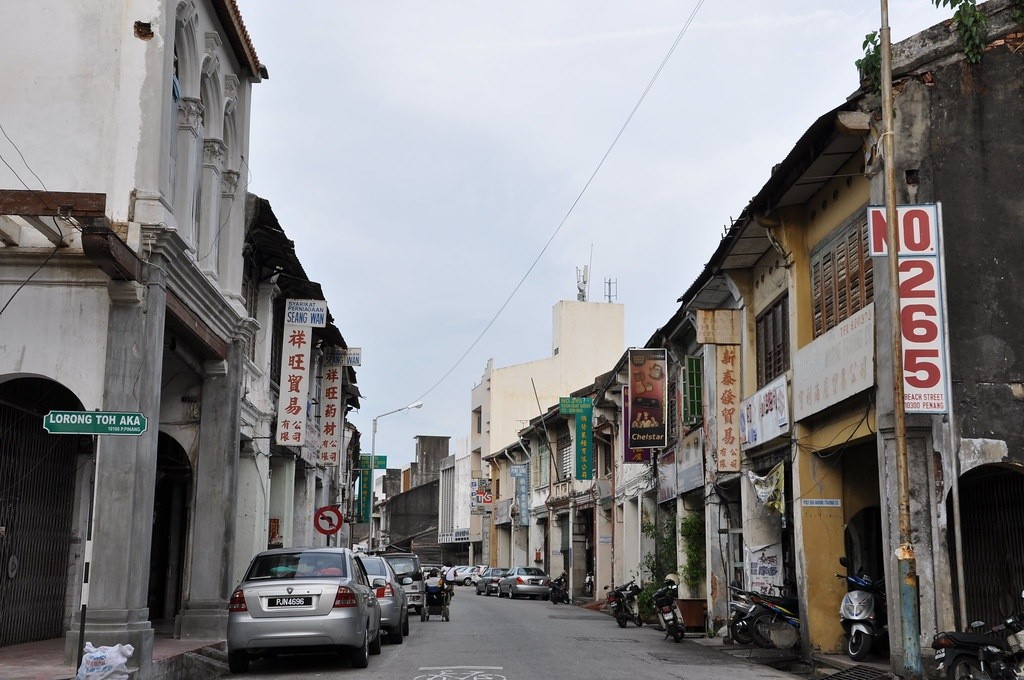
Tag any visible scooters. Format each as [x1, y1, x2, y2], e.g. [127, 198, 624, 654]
[834, 556, 886, 661]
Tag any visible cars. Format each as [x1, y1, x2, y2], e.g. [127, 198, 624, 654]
[226, 546, 382, 671]
[358, 555, 409, 645]
[476, 568, 510, 595]
[420, 562, 488, 587]
[497, 566, 551, 600]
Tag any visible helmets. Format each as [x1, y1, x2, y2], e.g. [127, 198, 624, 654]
[664, 573, 685, 586]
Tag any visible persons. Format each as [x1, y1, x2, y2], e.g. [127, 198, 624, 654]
[443, 562, 458, 591]
[424, 569, 448, 590]
[440, 563, 446, 573]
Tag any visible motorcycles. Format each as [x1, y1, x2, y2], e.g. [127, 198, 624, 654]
[646, 578, 686, 643]
[601, 579, 642, 628]
[729, 583, 799, 648]
[932, 614, 1023, 679]
[581, 569, 595, 597]
[546, 571, 570, 605]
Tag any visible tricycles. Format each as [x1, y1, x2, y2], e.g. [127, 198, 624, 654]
[420, 581, 453, 622]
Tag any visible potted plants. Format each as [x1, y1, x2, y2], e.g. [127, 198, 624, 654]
[671, 512, 707, 631]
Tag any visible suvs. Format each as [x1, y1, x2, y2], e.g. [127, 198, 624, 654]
[378, 551, 425, 615]
